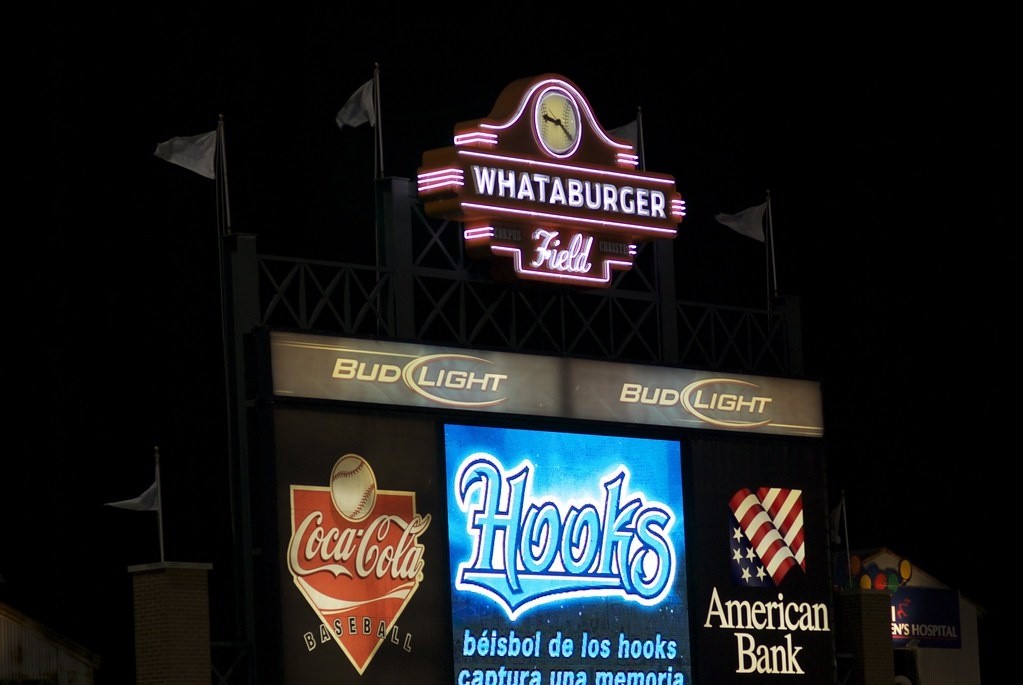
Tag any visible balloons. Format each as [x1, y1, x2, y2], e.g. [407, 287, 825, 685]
[850, 555, 912, 596]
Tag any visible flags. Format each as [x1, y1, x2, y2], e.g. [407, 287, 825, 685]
[715, 202, 768, 243]
[829, 503, 842, 544]
[104, 481, 159, 510]
[335, 78, 376, 130]
[153, 130, 216, 179]
[608, 120, 638, 152]
